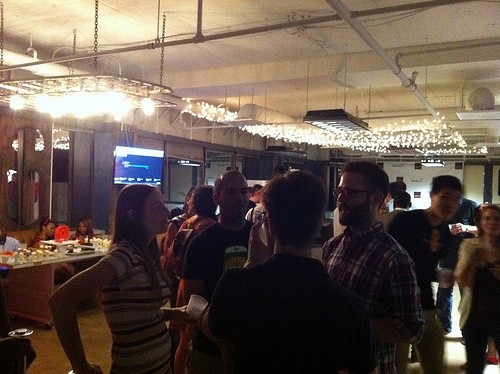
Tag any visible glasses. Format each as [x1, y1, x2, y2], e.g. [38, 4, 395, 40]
[332, 187, 367, 198]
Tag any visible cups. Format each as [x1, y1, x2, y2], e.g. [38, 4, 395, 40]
[439, 268, 451, 288]
[185, 294, 208, 320]
[85, 237, 112, 248]
[448, 224, 456, 234]
[17, 245, 59, 260]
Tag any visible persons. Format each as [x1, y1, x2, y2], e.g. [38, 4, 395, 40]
[49, 184, 202, 374]
[0, 161, 500, 374]
[201, 171, 377, 374]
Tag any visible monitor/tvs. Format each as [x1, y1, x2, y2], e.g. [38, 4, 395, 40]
[113, 145, 164, 187]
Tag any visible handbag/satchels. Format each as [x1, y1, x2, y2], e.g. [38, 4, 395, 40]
[163, 229, 194, 279]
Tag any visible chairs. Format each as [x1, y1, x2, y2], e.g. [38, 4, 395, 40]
[6, 228, 40, 248]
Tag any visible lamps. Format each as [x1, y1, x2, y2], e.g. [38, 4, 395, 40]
[0, 0, 445, 167]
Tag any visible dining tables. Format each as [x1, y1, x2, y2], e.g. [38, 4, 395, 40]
[0, 251, 113, 331]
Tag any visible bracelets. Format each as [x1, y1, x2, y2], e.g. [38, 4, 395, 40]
[465, 225, 470, 233]
